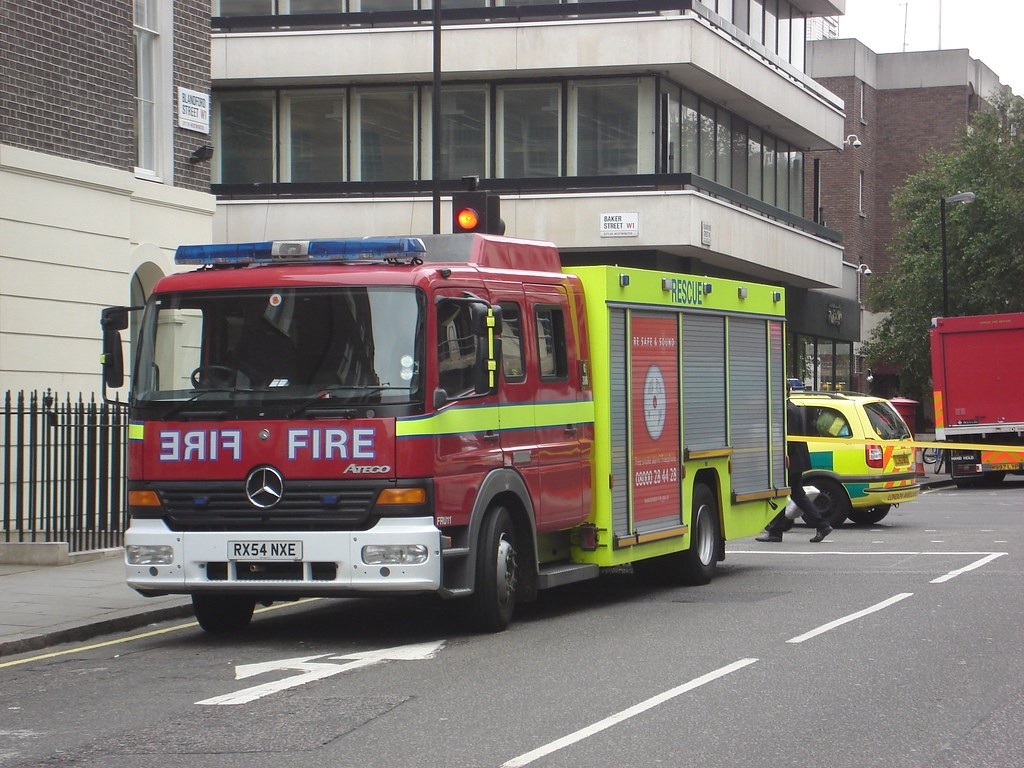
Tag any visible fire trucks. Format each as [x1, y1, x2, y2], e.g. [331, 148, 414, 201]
[924, 315, 1024, 487]
[96, 231, 793, 634]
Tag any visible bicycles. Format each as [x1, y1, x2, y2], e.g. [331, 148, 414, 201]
[922, 439, 947, 474]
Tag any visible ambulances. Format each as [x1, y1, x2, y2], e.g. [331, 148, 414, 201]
[780, 384, 923, 525]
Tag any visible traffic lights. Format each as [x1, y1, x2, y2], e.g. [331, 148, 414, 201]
[451, 192, 488, 234]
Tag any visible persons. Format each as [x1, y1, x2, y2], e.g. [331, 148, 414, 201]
[755, 379, 834, 543]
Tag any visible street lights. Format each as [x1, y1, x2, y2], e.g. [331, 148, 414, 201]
[938, 188, 977, 315]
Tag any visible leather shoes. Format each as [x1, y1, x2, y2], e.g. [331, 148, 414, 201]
[754, 535, 783, 543]
[810, 527, 833, 542]
